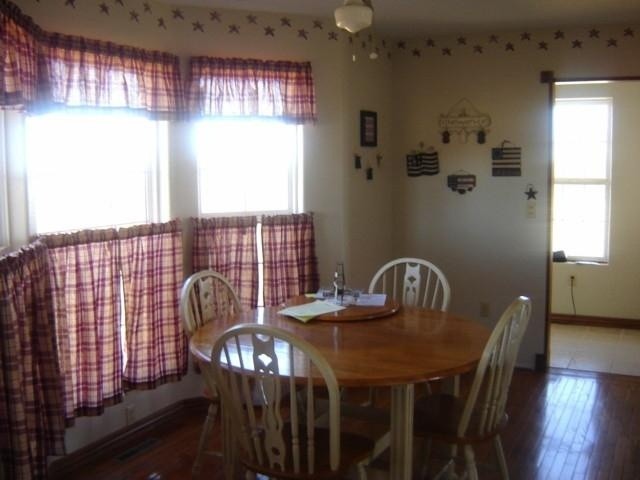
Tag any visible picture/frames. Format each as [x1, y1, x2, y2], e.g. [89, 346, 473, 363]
[360, 109, 378, 148]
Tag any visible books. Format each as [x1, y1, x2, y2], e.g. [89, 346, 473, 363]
[277, 299, 347, 324]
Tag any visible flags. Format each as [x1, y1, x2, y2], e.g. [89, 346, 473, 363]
[491, 146, 522, 177]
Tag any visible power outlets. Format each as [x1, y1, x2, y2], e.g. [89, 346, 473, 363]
[568, 271, 577, 287]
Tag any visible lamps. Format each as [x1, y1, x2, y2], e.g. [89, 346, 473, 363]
[333, 0, 374, 36]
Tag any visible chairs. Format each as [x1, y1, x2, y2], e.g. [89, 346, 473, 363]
[208, 321, 376, 480]
[412, 295, 533, 479]
[367, 258, 451, 412]
[177, 268, 293, 478]
